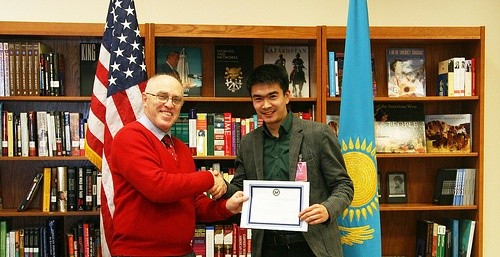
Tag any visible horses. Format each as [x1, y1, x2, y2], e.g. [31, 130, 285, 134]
[276, 61, 288, 74]
[290, 62, 306, 98]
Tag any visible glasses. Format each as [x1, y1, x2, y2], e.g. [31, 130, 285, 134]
[145, 91, 183, 104]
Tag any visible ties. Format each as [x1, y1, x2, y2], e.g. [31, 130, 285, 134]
[162, 135, 178, 162]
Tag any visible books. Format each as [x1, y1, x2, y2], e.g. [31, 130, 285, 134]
[215, 45, 254, 97]
[1, 110, 88, 157]
[328, 45, 476, 98]
[434, 168, 476, 206]
[325, 101, 472, 153]
[168, 108, 313, 156]
[385, 171, 408, 203]
[191, 162, 252, 257]
[416, 218, 476, 257]
[16, 166, 103, 213]
[0, 42, 100, 96]
[156, 44, 203, 96]
[263, 45, 310, 99]
[0, 217, 102, 257]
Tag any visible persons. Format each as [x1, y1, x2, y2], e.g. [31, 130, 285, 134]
[111, 73, 249, 257]
[214, 64, 354, 257]
[156, 51, 180, 81]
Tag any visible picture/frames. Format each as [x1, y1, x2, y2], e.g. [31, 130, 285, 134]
[377, 169, 383, 204]
[386, 171, 408, 203]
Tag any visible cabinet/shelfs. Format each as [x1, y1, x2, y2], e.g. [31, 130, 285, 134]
[0, 21, 486, 257]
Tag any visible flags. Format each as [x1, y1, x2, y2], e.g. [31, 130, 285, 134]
[336, 0, 382, 257]
[85, 0, 148, 257]
